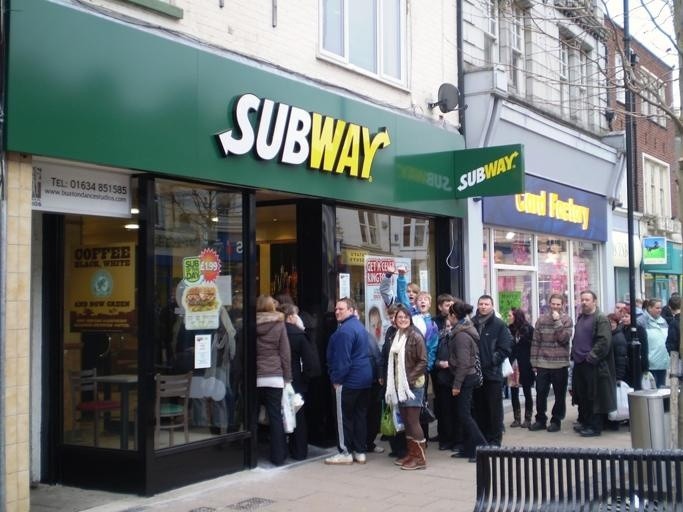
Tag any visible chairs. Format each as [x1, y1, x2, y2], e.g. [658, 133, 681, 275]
[69, 366, 122, 447]
[133, 370, 193, 451]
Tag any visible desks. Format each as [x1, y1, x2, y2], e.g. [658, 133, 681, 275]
[91, 375, 138, 449]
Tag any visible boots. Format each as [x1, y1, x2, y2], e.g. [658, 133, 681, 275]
[521, 406, 532, 428]
[396, 436, 427, 469]
[512, 408, 522, 427]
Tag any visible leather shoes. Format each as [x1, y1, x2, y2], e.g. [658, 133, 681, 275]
[531, 420, 547, 430]
[574, 421, 601, 436]
[441, 441, 478, 462]
[546, 421, 561, 432]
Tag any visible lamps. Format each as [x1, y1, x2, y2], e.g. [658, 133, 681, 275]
[130, 189, 140, 214]
[123, 210, 140, 229]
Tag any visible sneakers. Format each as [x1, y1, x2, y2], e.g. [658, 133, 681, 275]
[356, 451, 367, 463]
[324, 453, 354, 464]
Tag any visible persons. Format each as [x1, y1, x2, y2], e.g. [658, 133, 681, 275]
[570, 290, 618, 437]
[607, 300, 633, 321]
[528, 293, 573, 432]
[469, 294, 514, 449]
[619, 306, 650, 390]
[368, 306, 385, 354]
[439, 300, 491, 464]
[606, 314, 629, 432]
[507, 306, 535, 430]
[254, 294, 296, 465]
[440, 313, 461, 346]
[394, 266, 441, 448]
[663, 289, 682, 363]
[383, 307, 428, 471]
[376, 303, 426, 460]
[430, 291, 456, 333]
[633, 297, 643, 315]
[276, 305, 322, 461]
[325, 297, 378, 468]
[380, 261, 421, 311]
[660, 293, 682, 326]
[270, 274, 277, 296]
[634, 298, 671, 413]
